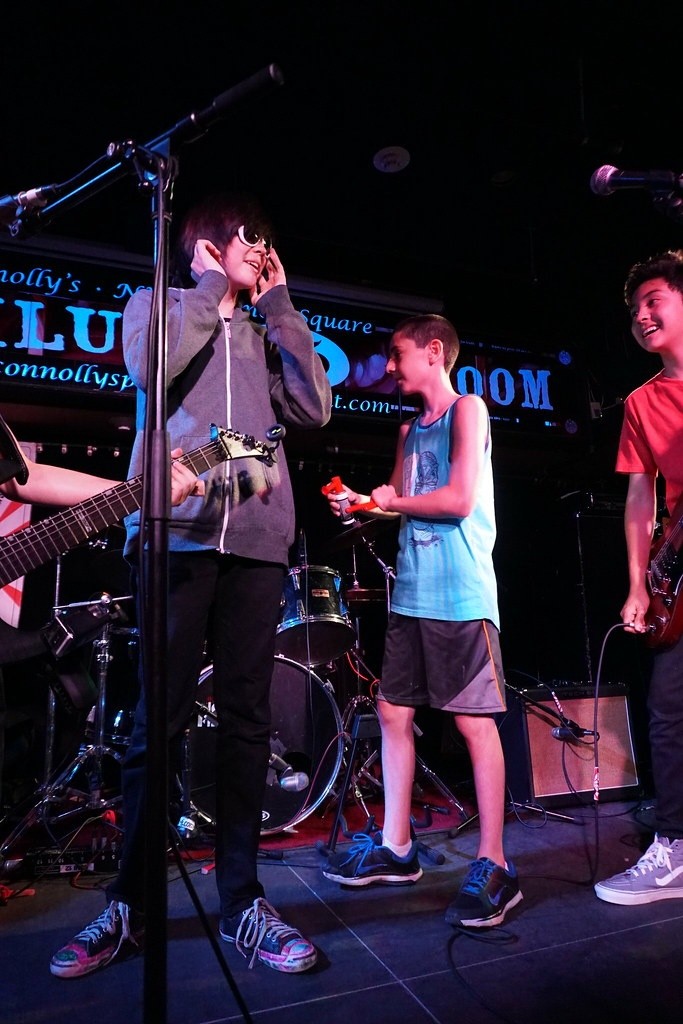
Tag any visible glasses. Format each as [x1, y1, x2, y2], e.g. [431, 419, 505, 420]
[235, 225, 272, 257]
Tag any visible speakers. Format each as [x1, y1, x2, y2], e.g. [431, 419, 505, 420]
[573, 513, 659, 797]
[507, 682, 647, 808]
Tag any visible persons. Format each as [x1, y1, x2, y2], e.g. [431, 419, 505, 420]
[594, 250, 683, 906]
[0, 414, 208, 515]
[47, 193, 320, 979]
[320, 315, 522, 929]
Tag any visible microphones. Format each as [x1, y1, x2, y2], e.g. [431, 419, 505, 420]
[590, 164, 683, 195]
[281, 768, 309, 791]
[266, 424, 287, 442]
[296, 529, 307, 562]
[0, 184, 60, 223]
[551, 726, 595, 741]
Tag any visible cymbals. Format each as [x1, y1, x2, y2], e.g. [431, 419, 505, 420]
[332, 516, 379, 542]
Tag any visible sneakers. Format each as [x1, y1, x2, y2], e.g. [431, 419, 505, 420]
[445, 858, 524, 927]
[218, 894, 318, 972]
[49, 900, 146, 976]
[322, 832, 424, 887]
[594, 833, 683, 906]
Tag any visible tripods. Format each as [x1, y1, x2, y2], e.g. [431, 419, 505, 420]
[164, 699, 283, 860]
[447, 677, 585, 840]
[0, 590, 137, 868]
[318, 603, 471, 826]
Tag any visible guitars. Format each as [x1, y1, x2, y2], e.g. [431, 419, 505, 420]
[0, 422, 287, 588]
[637, 491, 683, 652]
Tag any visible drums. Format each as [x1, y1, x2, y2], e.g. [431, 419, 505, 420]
[176, 655, 346, 834]
[275, 565, 356, 668]
